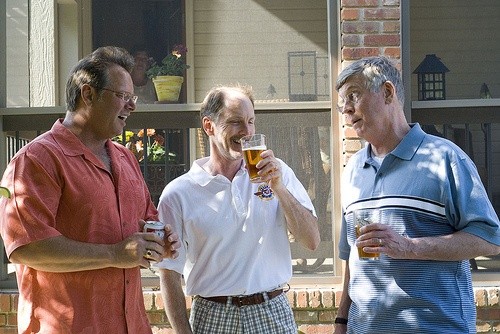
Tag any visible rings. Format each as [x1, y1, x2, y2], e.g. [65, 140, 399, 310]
[378, 238, 382, 246]
[147, 250, 152, 258]
[275, 162, 278, 170]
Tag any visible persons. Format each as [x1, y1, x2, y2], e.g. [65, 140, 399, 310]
[333, 56, 500, 334]
[150, 84, 320, 334]
[130, 46, 157, 104]
[0, 46, 182, 334]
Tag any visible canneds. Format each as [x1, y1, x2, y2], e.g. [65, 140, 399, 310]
[143, 221, 166, 260]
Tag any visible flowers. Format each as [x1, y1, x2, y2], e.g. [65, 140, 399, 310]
[146, 44, 190, 79]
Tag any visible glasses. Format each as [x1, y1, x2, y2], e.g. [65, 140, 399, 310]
[90, 85, 138, 103]
[336, 82, 384, 113]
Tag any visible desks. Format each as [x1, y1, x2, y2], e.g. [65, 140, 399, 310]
[412, 98, 500, 128]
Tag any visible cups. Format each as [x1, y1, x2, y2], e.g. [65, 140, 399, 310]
[240, 133, 267, 182]
[352, 209, 382, 261]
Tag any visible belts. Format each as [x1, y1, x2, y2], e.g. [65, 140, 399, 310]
[199, 289, 282, 307]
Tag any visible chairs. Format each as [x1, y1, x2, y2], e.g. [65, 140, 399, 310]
[195, 99, 333, 272]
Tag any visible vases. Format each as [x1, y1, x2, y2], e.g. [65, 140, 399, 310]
[150, 76, 185, 105]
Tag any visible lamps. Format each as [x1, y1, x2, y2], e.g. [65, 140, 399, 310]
[412, 53, 450, 102]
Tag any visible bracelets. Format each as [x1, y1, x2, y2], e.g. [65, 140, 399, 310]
[335, 318, 348, 325]
